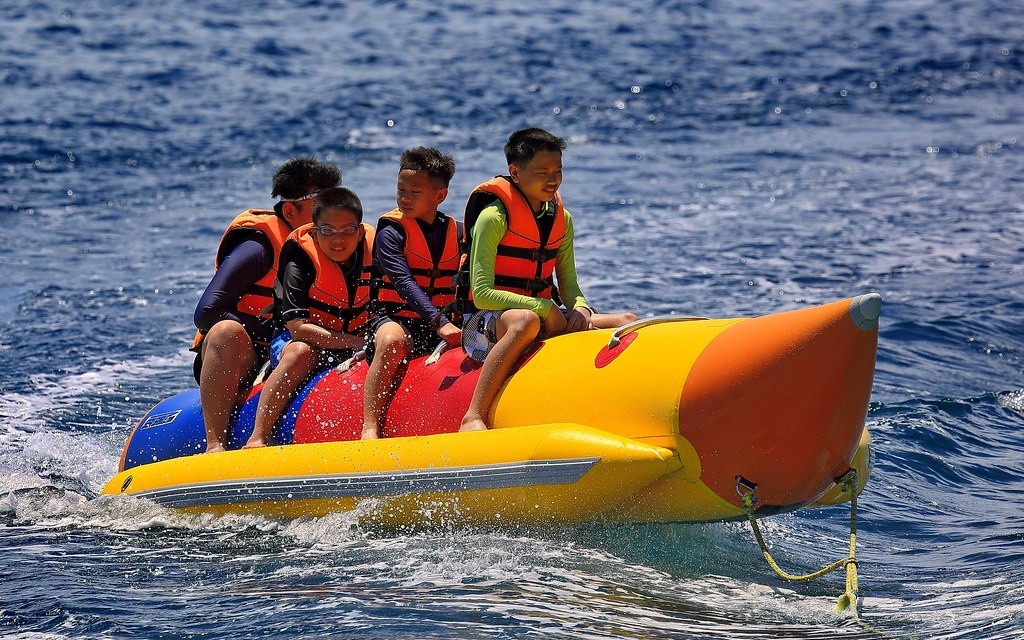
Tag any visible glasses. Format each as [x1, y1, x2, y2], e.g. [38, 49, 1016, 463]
[319, 224, 357, 236]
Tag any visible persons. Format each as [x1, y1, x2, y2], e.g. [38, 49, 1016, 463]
[456, 128, 638, 434]
[194, 158, 341, 454]
[240, 186, 376, 450]
[361, 148, 464, 441]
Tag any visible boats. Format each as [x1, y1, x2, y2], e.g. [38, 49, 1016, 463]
[98, 291, 883, 537]
